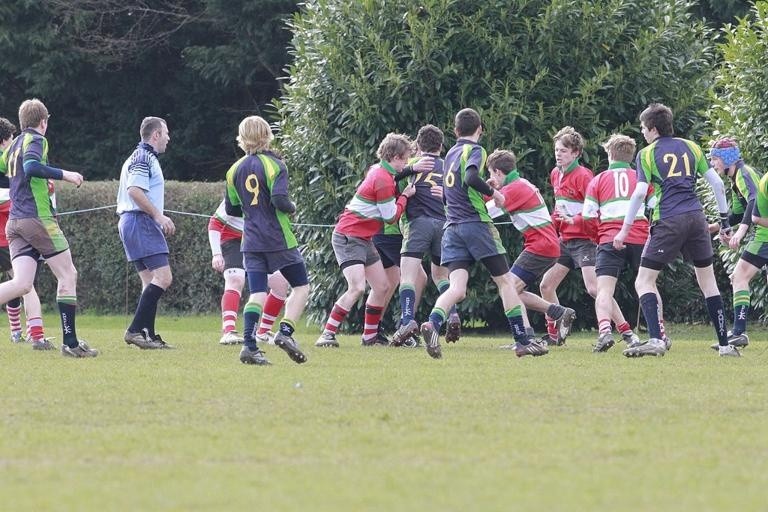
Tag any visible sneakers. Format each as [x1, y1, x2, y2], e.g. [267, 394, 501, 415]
[623, 338, 666, 357]
[712, 330, 750, 350]
[10, 326, 177, 360]
[535, 334, 564, 347]
[592, 333, 615, 353]
[220, 315, 464, 366]
[622, 330, 640, 347]
[641, 337, 672, 350]
[554, 307, 576, 346]
[719, 344, 741, 358]
[515, 341, 550, 357]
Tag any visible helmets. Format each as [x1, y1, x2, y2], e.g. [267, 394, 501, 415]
[711, 138, 740, 167]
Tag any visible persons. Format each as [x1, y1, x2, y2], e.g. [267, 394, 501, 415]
[315, 132, 416, 347]
[707, 137, 768, 350]
[0, 117, 58, 352]
[208, 198, 288, 346]
[536, 126, 640, 349]
[420, 108, 549, 359]
[613, 103, 740, 356]
[116, 116, 180, 350]
[582, 133, 672, 353]
[0, 97, 98, 358]
[751, 171, 768, 227]
[224, 115, 311, 366]
[481, 148, 576, 352]
[361, 124, 461, 347]
